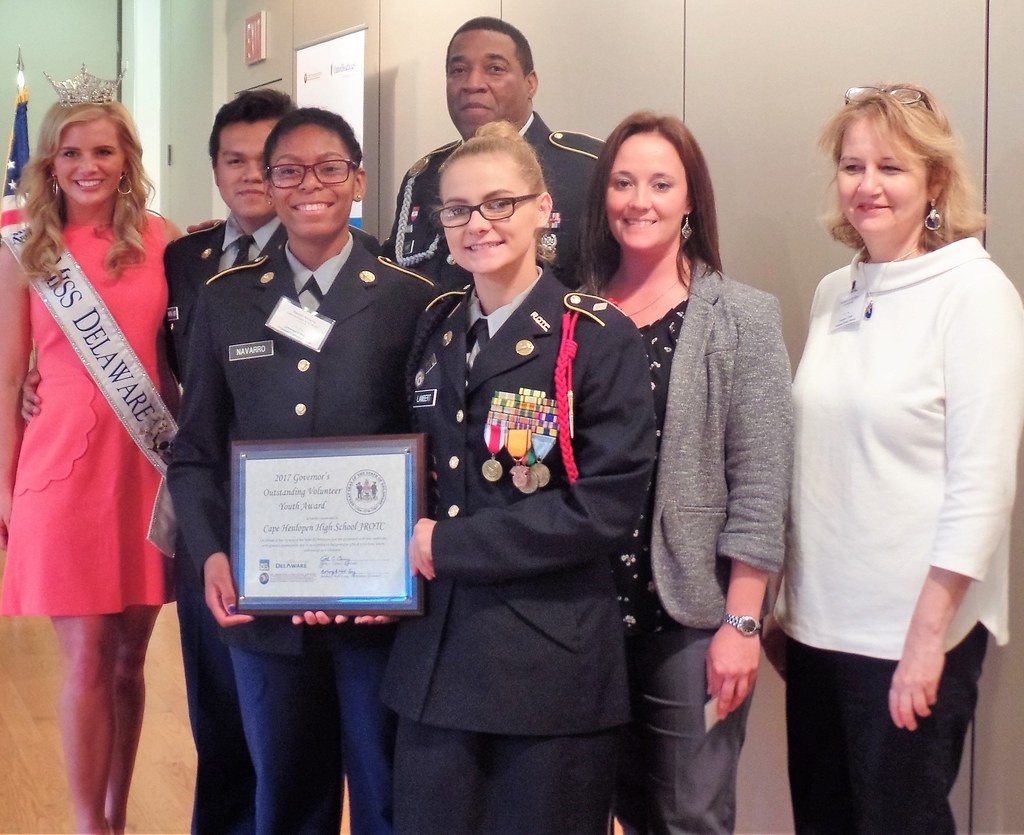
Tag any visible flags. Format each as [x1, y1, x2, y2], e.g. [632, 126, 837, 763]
[0, 90, 29, 245]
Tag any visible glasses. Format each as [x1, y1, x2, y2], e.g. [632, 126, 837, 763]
[264, 158, 359, 188]
[429, 192, 542, 229]
[844, 86, 934, 114]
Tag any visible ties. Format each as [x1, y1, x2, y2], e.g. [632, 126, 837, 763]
[232, 233, 255, 269]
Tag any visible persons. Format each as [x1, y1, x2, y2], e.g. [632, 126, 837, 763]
[769, 83, 1024, 835]
[21, 18, 790, 835]
[0, 64, 182, 835]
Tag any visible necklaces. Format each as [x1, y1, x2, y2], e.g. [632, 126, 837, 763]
[860, 244, 918, 317]
[627, 278, 683, 317]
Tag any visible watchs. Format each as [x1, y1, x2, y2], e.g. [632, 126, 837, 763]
[723, 612, 762, 635]
